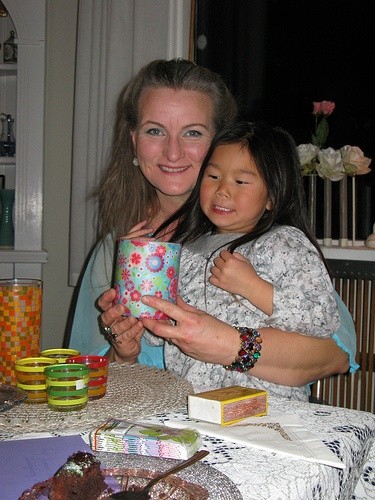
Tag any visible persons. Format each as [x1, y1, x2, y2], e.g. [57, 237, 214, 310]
[121, 119, 341, 402]
[66, 58, 358, 404]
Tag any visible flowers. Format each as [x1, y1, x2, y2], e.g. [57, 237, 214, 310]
[296, 101, 372, 181]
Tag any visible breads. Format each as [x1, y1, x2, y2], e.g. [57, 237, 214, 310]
[49, 451, 109, 500]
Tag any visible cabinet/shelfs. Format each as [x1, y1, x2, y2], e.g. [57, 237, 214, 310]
[0, 0, 45, 248]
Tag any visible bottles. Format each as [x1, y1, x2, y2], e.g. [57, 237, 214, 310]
[4, 31, 17, 64]
[0, 189, 14, 250]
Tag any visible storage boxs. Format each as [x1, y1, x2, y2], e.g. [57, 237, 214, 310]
[187, 386, 268, 426]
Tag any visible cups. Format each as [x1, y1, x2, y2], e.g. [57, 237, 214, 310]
[14, 348, 109, 412]
[113, 238, 183, 321]
[0, 278, 43, 389]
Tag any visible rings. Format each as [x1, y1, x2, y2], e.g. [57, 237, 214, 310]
[104, 326, 118, 338]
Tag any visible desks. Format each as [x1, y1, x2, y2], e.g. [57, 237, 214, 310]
[0, 361, 375, 500]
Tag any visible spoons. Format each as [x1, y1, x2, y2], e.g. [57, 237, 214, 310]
[99, 450, 210, 500]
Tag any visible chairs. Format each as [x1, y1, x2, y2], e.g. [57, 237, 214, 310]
[313, 257, 375, 412]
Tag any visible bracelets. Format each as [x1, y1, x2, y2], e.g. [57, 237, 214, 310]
[224, 326, 263, 372]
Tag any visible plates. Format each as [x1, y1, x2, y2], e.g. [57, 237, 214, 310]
[16, 467, 208, 500]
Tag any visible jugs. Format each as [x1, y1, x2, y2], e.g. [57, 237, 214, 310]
[0, 112, 16, 157]
